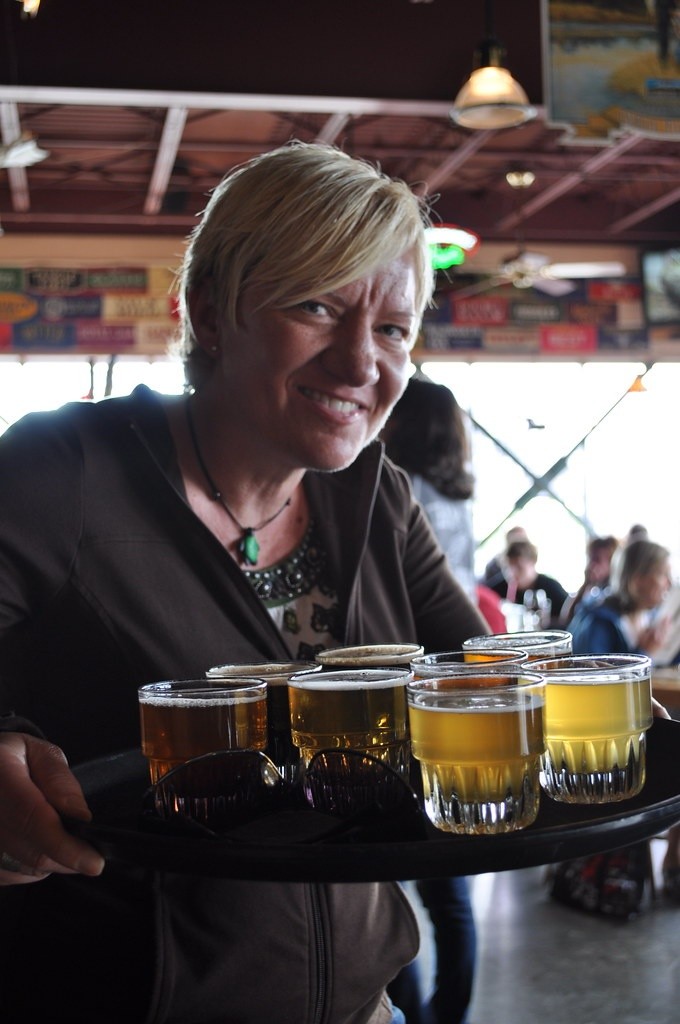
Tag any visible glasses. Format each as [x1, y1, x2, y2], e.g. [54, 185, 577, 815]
[142, 747, 426, 852]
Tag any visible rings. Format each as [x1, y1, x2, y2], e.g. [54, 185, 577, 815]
[1, 852, 21, 871]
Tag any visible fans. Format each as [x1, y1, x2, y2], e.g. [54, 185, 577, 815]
[457, 211, 626, 299]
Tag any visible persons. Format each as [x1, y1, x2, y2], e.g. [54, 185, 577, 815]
[559, 524, 680, 907]
[0, 140, 498, 1024]
[484, 527, 570, 631]
[383, 378, 477, 1024]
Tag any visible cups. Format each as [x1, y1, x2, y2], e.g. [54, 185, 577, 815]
[407, 675, 547, 835]
[138, 678, 268, 819]
[315, 643, 424, 672]
[287, 667, 414, 813]
[464, 630, 573, 656]
[407, 649, 528, 674]
[521, 653, 653, 804]
[205, 663, 323, 789]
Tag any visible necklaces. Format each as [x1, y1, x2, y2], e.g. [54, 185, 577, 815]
[182, 392, 292, 567]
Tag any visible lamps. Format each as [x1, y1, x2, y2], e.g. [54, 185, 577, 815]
[447, 0, 536, 133]
